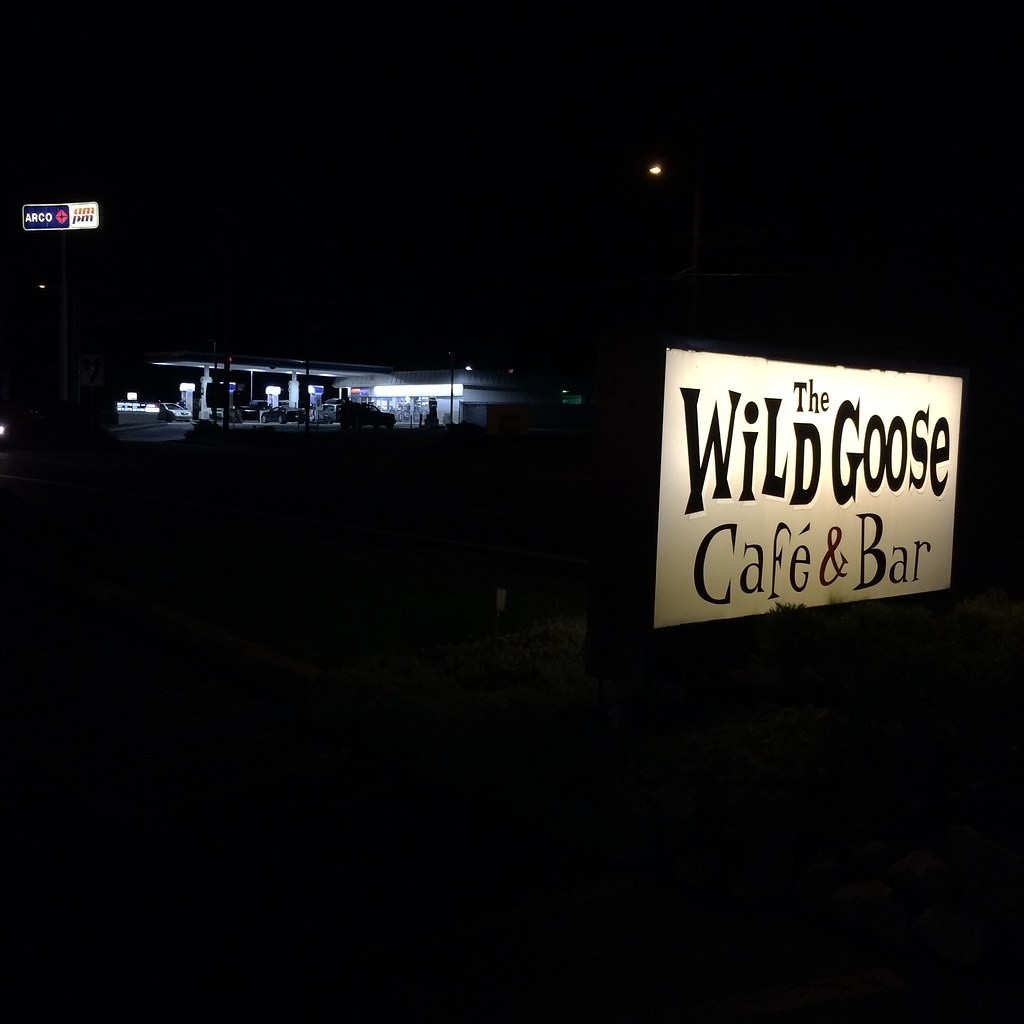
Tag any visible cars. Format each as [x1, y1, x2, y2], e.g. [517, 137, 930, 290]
[123, 383, 398, 428]
